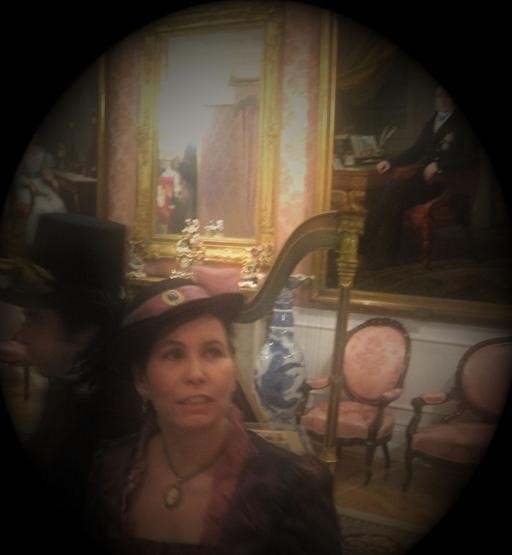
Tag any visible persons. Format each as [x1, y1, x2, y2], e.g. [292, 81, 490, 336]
[161, 141, 198, 234]
[0, 211, 133, 553]
[67, 277, 344, 555]
[351, 82, 466, 269]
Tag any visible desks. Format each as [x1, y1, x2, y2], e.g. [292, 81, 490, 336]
[334, 164, 417, 265]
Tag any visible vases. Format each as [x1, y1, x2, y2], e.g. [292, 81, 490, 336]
[255, 273, 314, 430]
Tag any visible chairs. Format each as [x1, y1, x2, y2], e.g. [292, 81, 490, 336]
[398, 169, 479, 272]
[300, 318, 411, 487]
[403, 336, 512, 498]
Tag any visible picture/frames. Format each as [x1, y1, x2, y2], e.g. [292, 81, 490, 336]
[15, 54, 109, 222]
[132, 0, 287, 267]
[301, 11, 512, 330]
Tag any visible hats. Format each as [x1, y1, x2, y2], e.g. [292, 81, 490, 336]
[110, 279, 243, 347]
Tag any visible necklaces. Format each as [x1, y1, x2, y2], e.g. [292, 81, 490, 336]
[158, 435, 232, 509]
